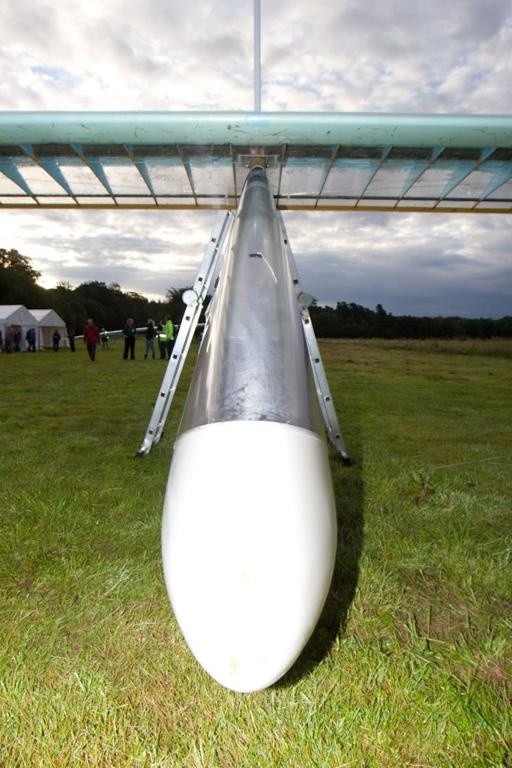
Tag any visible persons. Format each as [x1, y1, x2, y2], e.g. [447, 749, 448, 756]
[0, 316, 176, 362]
